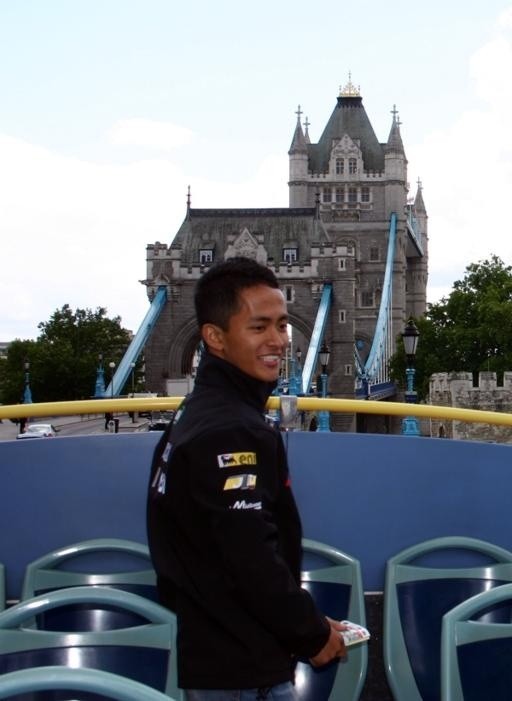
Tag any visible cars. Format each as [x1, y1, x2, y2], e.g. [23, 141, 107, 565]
[16, 424, 57, 440]
[148, 409, 177, 432]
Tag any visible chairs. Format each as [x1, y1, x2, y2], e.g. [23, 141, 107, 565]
[0, 534, 512, 701]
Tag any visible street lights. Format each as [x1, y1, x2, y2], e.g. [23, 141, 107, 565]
[401, 319, 421, 437]
[315, 339, 331, 433]
[23, 359, 33, 404]
[295, 346, 303, 389]
[95, 353, 136, 423]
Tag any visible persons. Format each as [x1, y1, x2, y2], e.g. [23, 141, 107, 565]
[147, 259, 346, 700]
